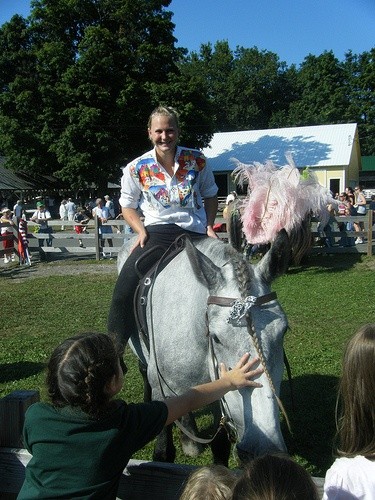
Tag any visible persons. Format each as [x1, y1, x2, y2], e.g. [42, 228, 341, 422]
[65, 198, 77, 220]
[31, 201, 53, 247]
[321, 324, 375, 500]
[179, 464, 239, 500]
[14, 199, 22, 217]
[232, 456, 321, 500]
[92, 198, 114, 259]
[226, 191, 237, 205]
[0, 208, 15, 264]
[17, 333, 264, 500]
[104, 195, 115, 219]
[317, 185, 366, 248]
[73, 207, 89, 247]
[369, 195, 375, 210]
[107, 105, 218, 372]
[60, 200, 67, 230]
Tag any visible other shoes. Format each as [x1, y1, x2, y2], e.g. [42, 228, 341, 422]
[80, 244, 83, 247]
[82, 230, 89, 234]
[355, 239, 363, 244]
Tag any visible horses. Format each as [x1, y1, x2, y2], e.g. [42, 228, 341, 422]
[117, 232, 290, 464]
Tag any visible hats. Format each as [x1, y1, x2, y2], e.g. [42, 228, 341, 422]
[36, 202, 43, 207]
[104, 195, 109, 199]
[1, 208, 10, 214]
